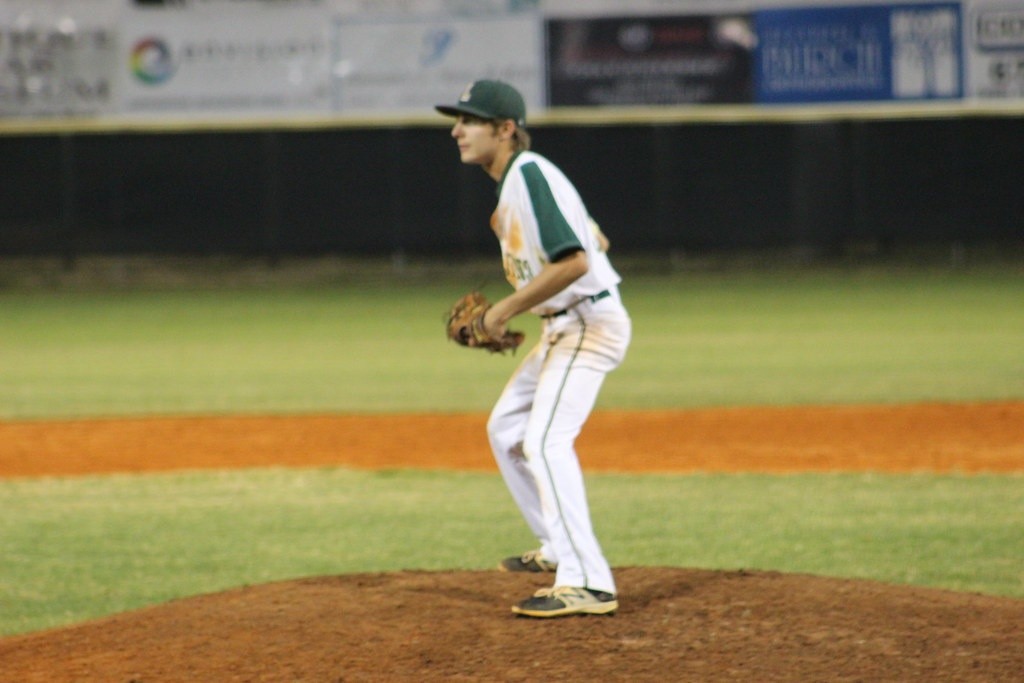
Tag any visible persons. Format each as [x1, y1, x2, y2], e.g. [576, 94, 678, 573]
[435, 79, 633, 617]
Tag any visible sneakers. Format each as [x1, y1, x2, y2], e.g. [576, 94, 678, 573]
[497, 550, 559, 575]
[511, 585, 619, 618]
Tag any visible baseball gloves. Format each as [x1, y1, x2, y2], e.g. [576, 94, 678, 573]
[442, 286, 526, 358]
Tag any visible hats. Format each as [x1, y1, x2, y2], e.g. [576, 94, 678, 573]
[435, 79, 528, 128]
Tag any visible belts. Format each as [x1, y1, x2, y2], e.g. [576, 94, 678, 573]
[540, 289, 610, 319]
